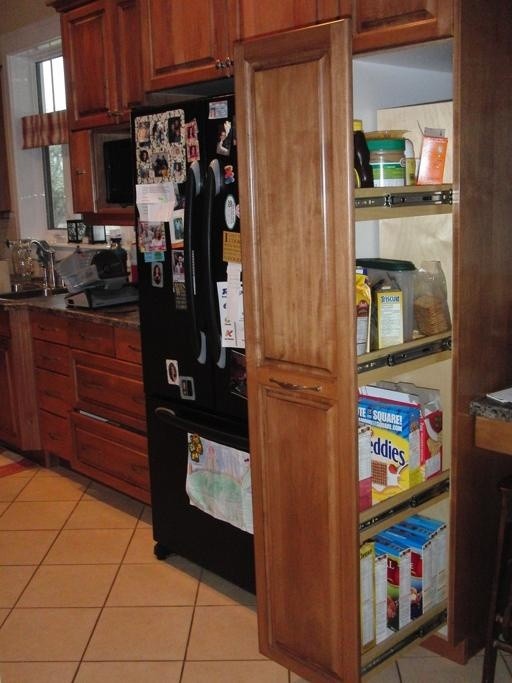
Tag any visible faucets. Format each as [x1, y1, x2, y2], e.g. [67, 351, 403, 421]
[30, 239, 57, 289]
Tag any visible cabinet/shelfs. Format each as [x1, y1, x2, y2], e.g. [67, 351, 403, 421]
[1, 304, 151, 504]
[233, 19, 510, 682]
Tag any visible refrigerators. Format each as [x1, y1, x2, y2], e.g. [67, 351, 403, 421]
[129, 96, 257, 597]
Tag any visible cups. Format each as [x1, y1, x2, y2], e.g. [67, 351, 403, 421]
[110, 230, 122, 243]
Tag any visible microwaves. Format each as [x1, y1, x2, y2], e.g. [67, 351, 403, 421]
[101, 137, 136, 206]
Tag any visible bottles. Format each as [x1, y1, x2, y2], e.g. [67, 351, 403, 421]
[353, 120, 374, 188]
[366, 139, 406, 187]
[129, 239, 139, 284]
[8, 238, 34, 277]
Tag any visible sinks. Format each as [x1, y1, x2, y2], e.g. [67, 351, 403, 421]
[0, 287, 69, 303]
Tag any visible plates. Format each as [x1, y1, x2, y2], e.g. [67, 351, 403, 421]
[364, 130, 410, 140]
